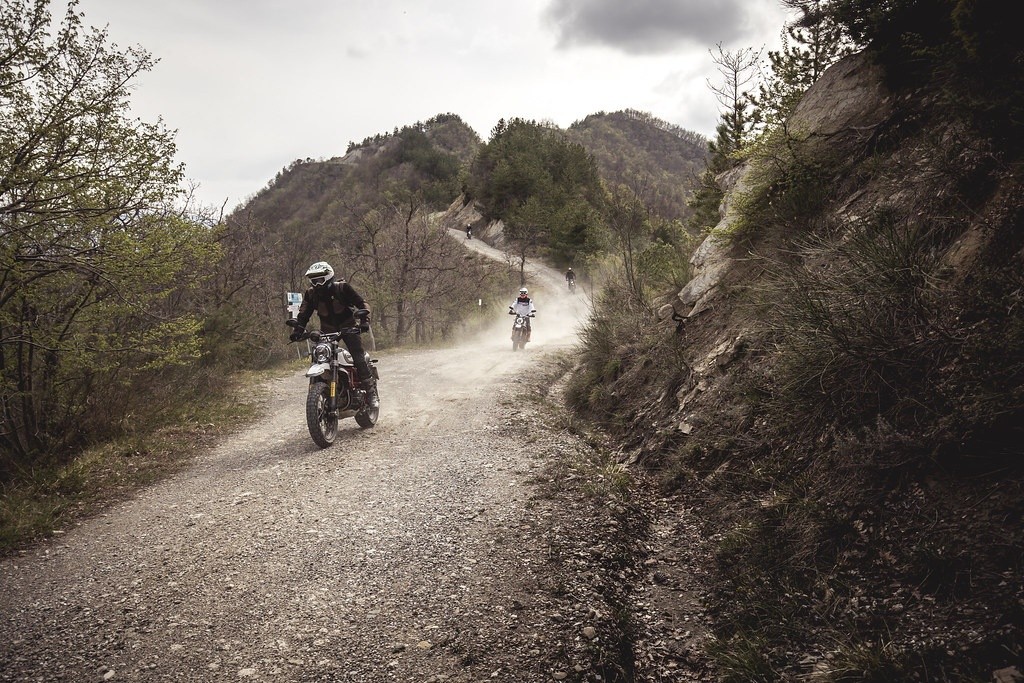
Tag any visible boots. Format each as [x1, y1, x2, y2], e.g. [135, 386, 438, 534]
[362, 376, 380, 410]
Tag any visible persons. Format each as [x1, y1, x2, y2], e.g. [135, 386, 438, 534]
[290, 262, 379, 408]
[467, 224, 472, 237]
[566, 268, 576, 290]
[509, 288, 535, 342]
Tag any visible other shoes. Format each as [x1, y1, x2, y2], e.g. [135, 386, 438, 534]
[526, 333, 531, 342]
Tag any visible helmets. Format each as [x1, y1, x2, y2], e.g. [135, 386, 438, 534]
[305, 262, 334, 287]
[568, 268, 572, 272]
[520, 288, 528, 295]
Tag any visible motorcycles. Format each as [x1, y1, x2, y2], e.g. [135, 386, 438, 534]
[566, 277, 576, 296]
[509, 306, 536, 352]
[284, 309, 381, 448]
[467, 229, 472, 240]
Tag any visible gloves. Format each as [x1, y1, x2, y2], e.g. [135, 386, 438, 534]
[290, 329, 302, 342]
[356, 316, 370, 332]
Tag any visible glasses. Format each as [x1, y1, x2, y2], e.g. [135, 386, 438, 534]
[311, 276, 326, 286]
[521, 293, 525, 294]
